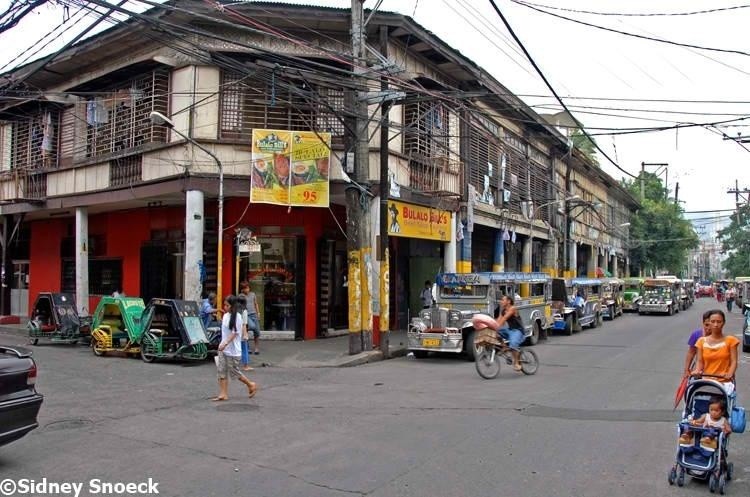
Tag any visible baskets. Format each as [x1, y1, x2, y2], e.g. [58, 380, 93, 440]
[474, 327, 497, 346]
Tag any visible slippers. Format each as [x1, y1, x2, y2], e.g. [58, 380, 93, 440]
[212, 395, 227, 401]
[249, 383, 256, 398]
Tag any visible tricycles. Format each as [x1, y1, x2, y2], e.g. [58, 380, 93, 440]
[26, 292, 91, 347]
[88, 292, 224, 365]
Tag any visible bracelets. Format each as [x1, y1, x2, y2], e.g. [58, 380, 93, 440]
[222, 341, 228, 347]
[258, 311, 261, 314]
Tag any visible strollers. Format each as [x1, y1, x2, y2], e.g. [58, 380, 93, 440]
[667, 373, 733, 496]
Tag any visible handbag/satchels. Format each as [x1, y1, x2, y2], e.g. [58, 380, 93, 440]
[731, 407, 746, 433]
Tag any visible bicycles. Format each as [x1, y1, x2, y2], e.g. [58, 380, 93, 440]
[474, 328, 539, 378]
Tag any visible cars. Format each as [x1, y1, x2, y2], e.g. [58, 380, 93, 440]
[696, 276, 750, 355]
[0, 345, 43, 447]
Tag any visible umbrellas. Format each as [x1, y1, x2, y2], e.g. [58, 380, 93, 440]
[672, 358, 695, 412]
[597, 267, 612, 276]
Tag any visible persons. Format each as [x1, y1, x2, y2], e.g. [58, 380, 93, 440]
[677, 397, 730, 443]
[389, 204, 400, 233]
[238, 281, 263, 355]
[236, 294, 254, 370]
[391, 171, 398, 190]
[715, 282, 744, 311]
[682, 311, 710, 378]
[201, 293, 225, 328]
[495, 295, 523, 371]
[419, 280, 432, 309]
[212, 295, 260, 401]
[692, 310, 739, 390]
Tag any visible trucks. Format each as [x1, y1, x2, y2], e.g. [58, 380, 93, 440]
[549, 274, 694, 336]
[404, 270, 552, 361]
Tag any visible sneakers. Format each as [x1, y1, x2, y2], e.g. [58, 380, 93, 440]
[513, 364, 521, 371]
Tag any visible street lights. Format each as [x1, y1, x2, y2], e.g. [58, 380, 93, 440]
[528, 194, 581, 270]
[594, 220, 630, 274]
[149, 110, 224, 323]
[565, 200, 603, 271]
